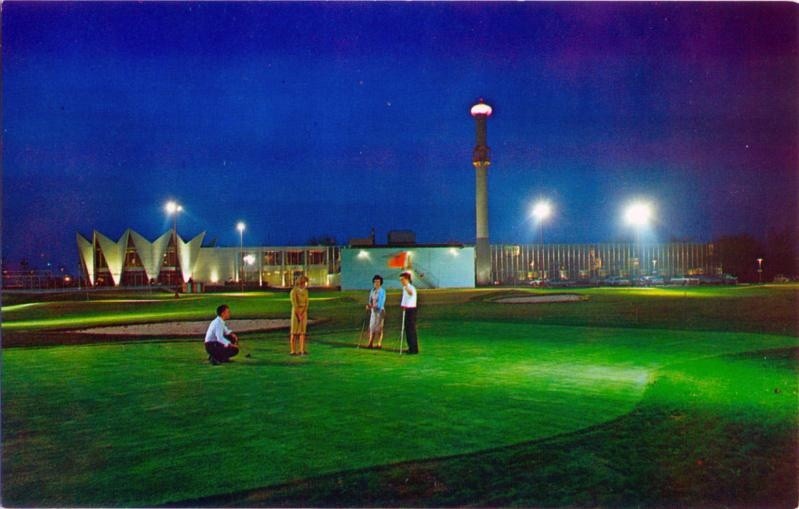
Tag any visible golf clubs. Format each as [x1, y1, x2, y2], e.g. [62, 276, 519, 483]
[399, 306, 406, 357]
[355, 303, 368, 351]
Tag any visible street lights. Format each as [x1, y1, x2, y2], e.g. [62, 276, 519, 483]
[532, 202, 551, 250]
[471, 97, 493, 287]
[237, 222, 247, 292]
[167, 201, 181, 246]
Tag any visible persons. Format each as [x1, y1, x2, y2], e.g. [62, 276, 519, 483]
[204, 304, 240, 366]
[399, 272, 419, 355]
[366, 275, 386, 349]
[290, 276, 310, 356]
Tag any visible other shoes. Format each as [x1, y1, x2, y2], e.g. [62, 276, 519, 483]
[288, 352, 296, 355]
[376, 345, 381, 349]
[367, 344, 372, 349]
[404, 349, 418, 354]
[209, 356, 220, 366]
[299, 351, 304, 355]
[220, 358, 230, 363]
